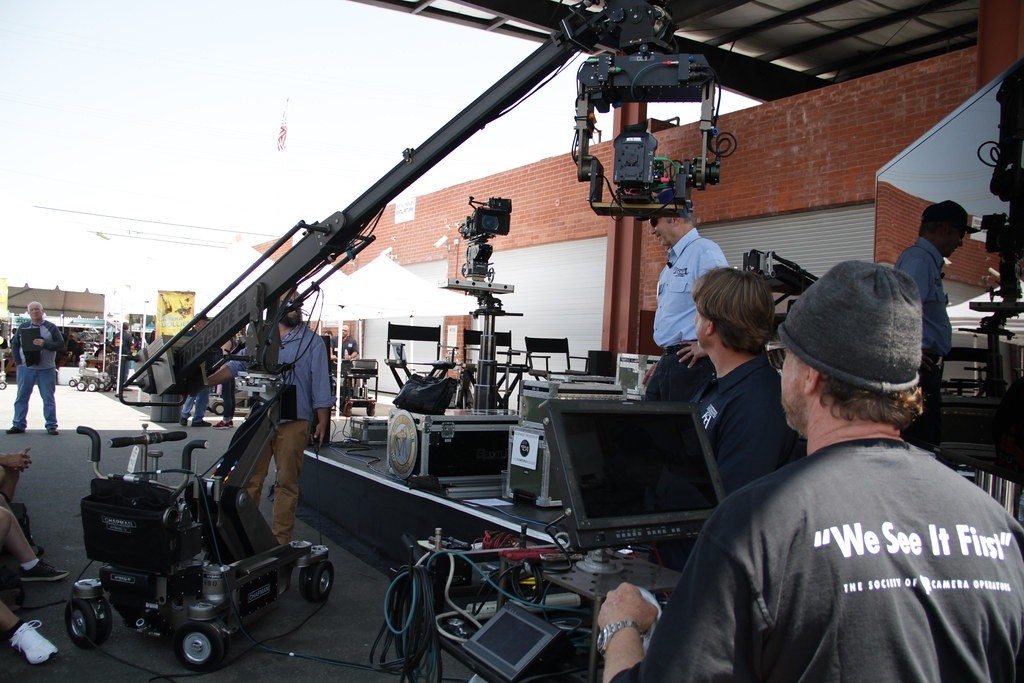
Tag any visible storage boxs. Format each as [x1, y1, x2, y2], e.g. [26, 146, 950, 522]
[348, 353, 662, 507]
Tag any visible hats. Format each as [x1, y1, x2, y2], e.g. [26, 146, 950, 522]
[778, 260, 922, 393]
[921, 200, 981, 234]
[635, 188, 690, 221]
[194, 313, 211, 321]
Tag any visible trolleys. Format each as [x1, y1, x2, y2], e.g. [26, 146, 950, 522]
[65, 424, 334, 670]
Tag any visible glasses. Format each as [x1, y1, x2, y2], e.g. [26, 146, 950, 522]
[649, 212, 680, 227]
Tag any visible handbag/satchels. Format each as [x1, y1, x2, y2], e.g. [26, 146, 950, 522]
[392, 374, 457, 415]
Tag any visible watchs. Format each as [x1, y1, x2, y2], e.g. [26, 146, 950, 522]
[596, 620, 643, 654]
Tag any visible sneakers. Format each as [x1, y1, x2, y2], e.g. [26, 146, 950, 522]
[229, 421, 233, 428]
[9, 619, 59, 666]
[180, 417, 187, 426]
[212, 420, 229, 429]
[191, 418, 211, 427]
[7, 426, 25, 434]
[19, 558, 70, 581]
[48, 428, 59, 435]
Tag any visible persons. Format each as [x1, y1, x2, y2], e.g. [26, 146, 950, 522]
[649, 268, 796, 570]
[643, 196, 731, 405]
[0, 289, 330, 663]
[891, 201, 979, 453]
[595, 259, 1024, 683]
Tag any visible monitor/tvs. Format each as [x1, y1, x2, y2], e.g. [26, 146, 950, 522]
[538, 398, 727, 545]
[464, 601, 563, 682]
